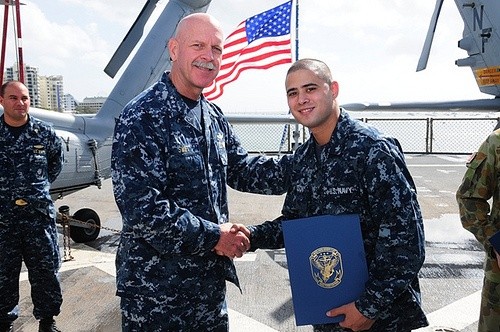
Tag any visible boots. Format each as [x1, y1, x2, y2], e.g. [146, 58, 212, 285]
[0, 322, 13, 332]
[39, 319, 61, 332]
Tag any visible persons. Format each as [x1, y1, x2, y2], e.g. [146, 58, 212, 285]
[110, 13, 294, 332]
[211, 59, 430, 332]
[0, 81, 63, 332]
[456, 128, 500, 332]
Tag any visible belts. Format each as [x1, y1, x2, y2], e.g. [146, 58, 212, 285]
[14, 199, 29, 206]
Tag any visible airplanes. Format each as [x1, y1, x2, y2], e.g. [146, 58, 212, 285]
[1, 0, 213, 244]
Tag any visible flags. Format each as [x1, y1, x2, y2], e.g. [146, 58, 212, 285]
[202, 0, 293, 101]
